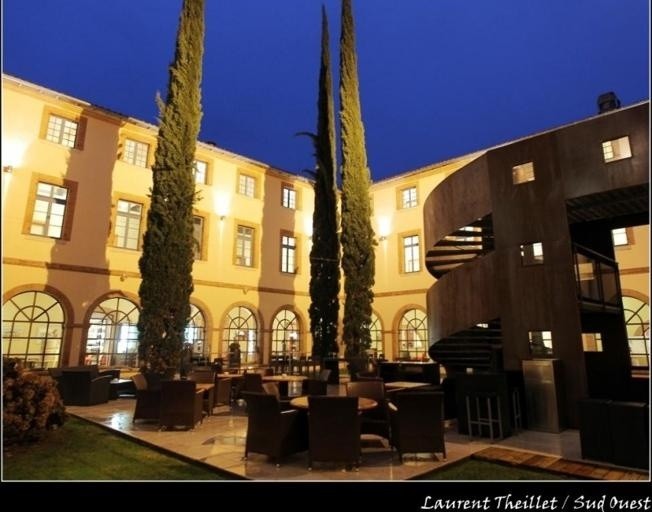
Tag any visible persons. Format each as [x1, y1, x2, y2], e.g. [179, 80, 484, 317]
[228, 335, 242, 375]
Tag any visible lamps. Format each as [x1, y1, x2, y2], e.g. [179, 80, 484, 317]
[4, 165, 14, 173]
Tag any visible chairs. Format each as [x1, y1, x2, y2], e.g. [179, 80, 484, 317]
[48, 350, 447, 472]
